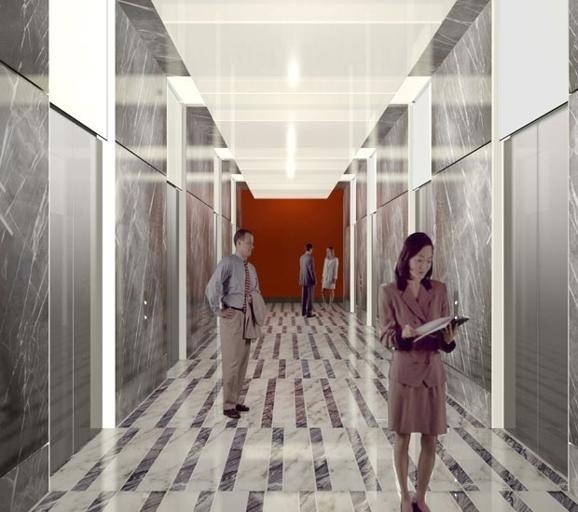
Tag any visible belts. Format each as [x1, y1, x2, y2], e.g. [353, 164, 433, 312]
[226, 306, 243, 311]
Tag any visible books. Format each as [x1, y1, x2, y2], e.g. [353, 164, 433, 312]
[413, 315, 470, 344]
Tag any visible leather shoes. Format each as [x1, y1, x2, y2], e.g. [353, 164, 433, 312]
[224, 404, 249, 418]
[302, 313, 315, 317]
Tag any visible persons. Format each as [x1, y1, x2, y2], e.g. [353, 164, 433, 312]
[320, 245, 339, 312]
[298, 242, 318, 318]
[377, 230, 459, 512]
[205, 228, 268, 419]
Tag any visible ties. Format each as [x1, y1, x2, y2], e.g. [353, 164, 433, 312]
[243, 261, 251, 312]
